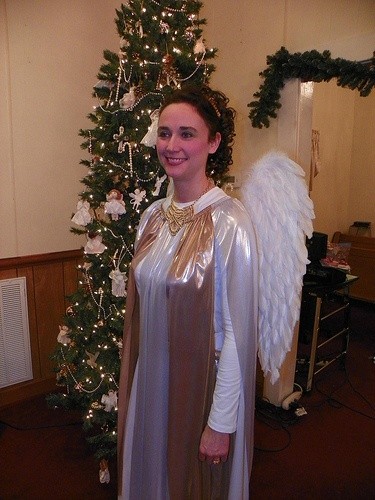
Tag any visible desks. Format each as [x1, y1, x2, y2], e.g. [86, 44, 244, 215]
[301, 268, 359, 396]
[328, 228, 375, 310]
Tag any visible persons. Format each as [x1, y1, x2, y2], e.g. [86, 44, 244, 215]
[114, 84, 260, 500]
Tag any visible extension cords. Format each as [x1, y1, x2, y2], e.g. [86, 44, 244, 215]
[281, 391, 302, 410]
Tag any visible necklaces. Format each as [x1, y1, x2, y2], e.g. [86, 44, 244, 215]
[166, 179, 211, 236]
